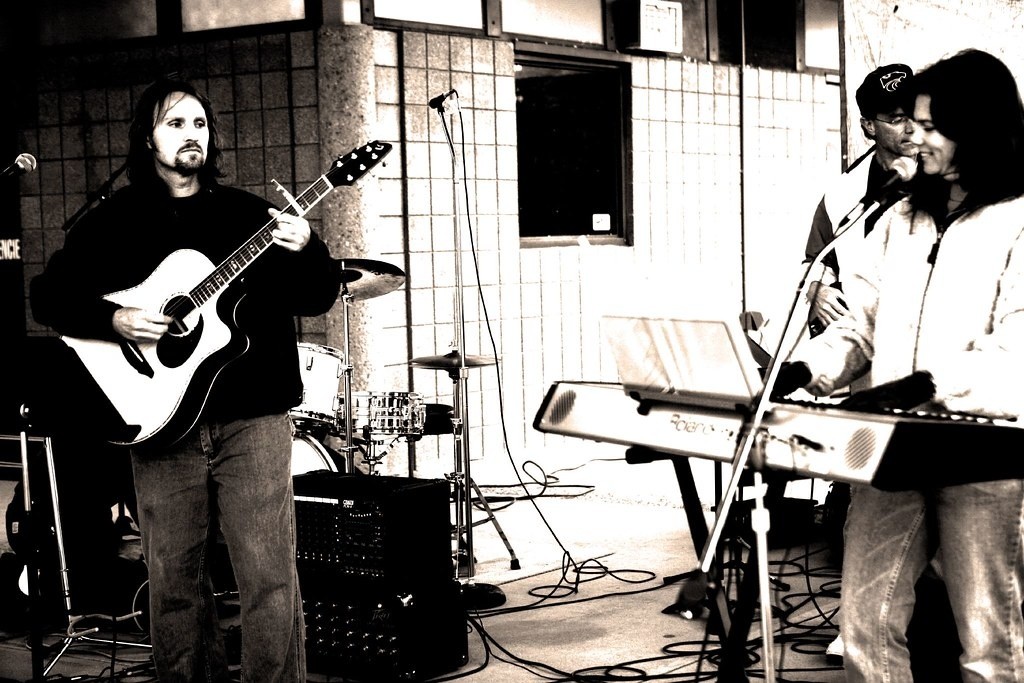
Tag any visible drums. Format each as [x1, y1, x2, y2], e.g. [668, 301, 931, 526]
[288, 340, 345, 430]
[289, 434, 366, 479]
[332, 389, 427, 438]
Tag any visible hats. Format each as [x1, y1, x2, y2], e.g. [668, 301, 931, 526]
[854, 64, 914, 119]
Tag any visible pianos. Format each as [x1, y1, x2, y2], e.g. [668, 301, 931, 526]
[530, 376, 1024, 497]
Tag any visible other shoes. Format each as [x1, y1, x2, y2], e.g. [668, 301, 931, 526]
[824, 633, 845, 667]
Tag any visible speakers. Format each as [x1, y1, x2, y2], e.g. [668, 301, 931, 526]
[292, 469, 468, 683]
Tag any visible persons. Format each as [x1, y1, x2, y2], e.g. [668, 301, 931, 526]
[29, 72, 339, 683]
[803, 48, 1024, 683]
[805, 63, 953, 662]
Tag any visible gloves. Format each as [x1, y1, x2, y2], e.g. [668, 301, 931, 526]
[840, 371, 936, 413]
[751, 361, 813, 404]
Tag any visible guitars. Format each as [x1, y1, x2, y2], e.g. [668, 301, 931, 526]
[52, 138, 394, 458]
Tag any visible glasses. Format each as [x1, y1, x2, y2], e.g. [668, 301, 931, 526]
[875, 115, 910, 127]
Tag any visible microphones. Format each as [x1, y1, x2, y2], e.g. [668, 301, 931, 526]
[429, 89, 455, 108]
[838, 157, 917, 229]
[0, 153, 36, 185]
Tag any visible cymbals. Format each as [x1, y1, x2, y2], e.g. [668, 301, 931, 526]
[331, 257, 405, 304]
[408, 351, 502, 373]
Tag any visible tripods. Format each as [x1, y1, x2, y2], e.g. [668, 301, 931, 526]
[445, 370, 522, 572]
[41, 436, 153, 678]
